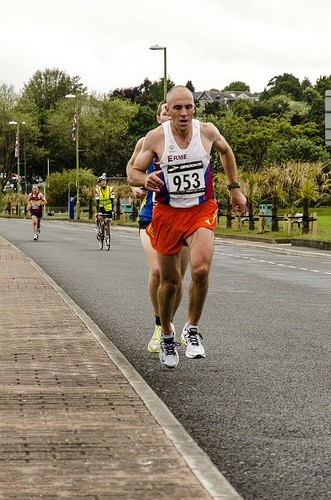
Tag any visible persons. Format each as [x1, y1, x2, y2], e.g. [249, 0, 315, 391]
[125, 86, 245, 369]
[94, 175, 115, 247]
[27, 185, 48, 241]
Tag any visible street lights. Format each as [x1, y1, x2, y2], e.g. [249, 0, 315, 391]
[149, 44, 168, 101]
[65, 93, 81, 222]
[10, 120, 28, 220]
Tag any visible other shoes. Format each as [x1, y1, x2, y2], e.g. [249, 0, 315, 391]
[34, 231, 39, 240]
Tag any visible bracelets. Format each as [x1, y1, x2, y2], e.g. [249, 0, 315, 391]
[227, 182, 241, 191]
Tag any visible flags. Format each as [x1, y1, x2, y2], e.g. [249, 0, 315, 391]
[72, 111, 76, 141]
[15, 134, 19, 158]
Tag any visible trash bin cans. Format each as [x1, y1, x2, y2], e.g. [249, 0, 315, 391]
[121, 204, 133, 220]
[259, 204, 273, 232]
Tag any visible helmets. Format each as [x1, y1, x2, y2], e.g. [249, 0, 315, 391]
[97, 176, 108, 183]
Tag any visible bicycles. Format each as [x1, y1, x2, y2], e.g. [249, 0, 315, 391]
[95, 213, 118, 251]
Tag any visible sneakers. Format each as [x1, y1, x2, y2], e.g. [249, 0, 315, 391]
[181, 322, 206, 359]
[148, 323, 162, 353]
[158, 323, 180, 369]
